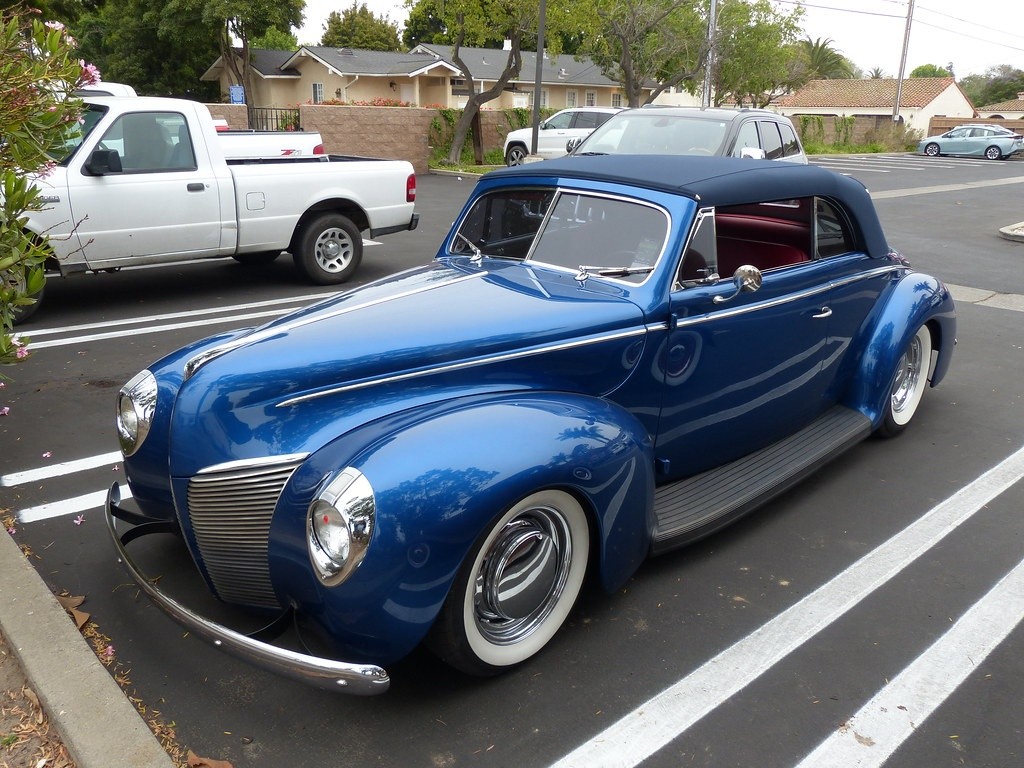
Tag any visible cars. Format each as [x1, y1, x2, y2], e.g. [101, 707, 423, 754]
[104, 155, 959, 675]
[919, 123, 1024, 160]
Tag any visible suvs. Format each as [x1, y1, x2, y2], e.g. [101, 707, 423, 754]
[503, 106, 632, 167]
[501, 103, 809, 259]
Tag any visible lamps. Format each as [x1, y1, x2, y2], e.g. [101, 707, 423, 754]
[390, 79, 398, 93]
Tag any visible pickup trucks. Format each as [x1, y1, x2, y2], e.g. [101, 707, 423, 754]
[154, 114, 230, 148]
[0, 97, 420, 328]
[0, 82, 325, 160]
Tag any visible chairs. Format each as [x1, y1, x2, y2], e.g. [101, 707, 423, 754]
[168, 125, 196, 170]
[123, 124, 176, 169]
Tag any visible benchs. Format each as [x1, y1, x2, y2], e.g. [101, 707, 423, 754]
[714, 213, 812, 280]
